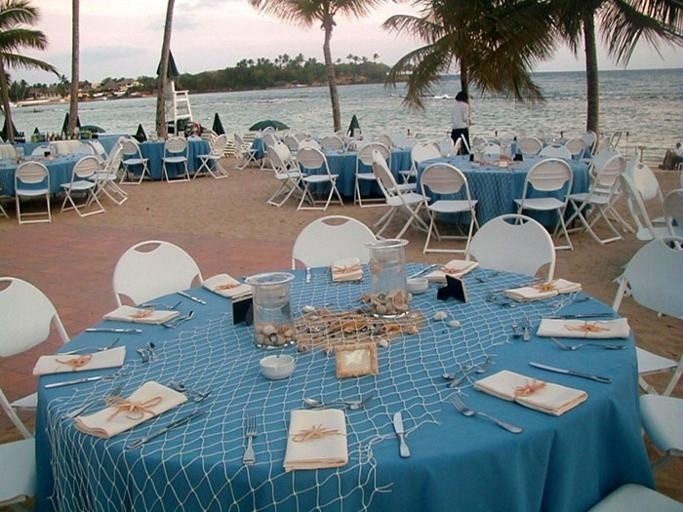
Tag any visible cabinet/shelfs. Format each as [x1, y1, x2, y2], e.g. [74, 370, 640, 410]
[165, 78, 194, 136]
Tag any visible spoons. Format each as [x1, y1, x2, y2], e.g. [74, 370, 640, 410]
[171, 379, 212, 400]
[442, 362, 478, 379]
[146, 341, 158, 362]
[510, 321, 523, 337]
[301, 396, 362, 407]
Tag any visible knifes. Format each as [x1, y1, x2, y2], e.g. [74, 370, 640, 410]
[393, 411, 411, 458]
[43, 376, 101, 388]
[85, 327, 142, 333]
[177, 290, 208, 304]
[306, 266, 311, 283]
[527, 360, 613, 382]
[543, 312, 612, 319]
[408, 263, 436, 278]
[126, 411, 203, 450]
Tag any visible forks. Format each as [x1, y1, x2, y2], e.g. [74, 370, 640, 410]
[448, 356, 494, 388]
[67, 387, 122, 418]
[59, 338, 119, 356]
[450, 393, 523, 433]
[522, 314, 531, 341]
[142, 297, 183, 310]
[243, 414, 258, 465]
[550, 337, 617, 351]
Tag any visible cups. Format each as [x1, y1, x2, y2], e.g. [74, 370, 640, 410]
[367, 239, 410, 319]
[242, 271, 296, 349]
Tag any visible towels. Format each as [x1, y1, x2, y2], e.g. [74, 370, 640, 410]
[103, 305, 180, 325]
[32, 344, 128, 377]
[283, 408, 349, 476]
[537, 318, 632, 338]
[76, 379, 190, 440]
[475, 368, 588, 417]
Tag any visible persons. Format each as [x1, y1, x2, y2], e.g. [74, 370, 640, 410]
[450, 91, 470, 155]
[671, 143, 683, 170]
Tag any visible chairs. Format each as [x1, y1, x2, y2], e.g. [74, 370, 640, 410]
[0, 277, 71, 439]
[589, 483, 683, 512]
[613, 236, 683, 436]
[0, 439, 37, 511]
[638, 394, 682, 471]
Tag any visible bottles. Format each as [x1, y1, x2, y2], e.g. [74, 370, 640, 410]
[13, 127, 98, 144]
